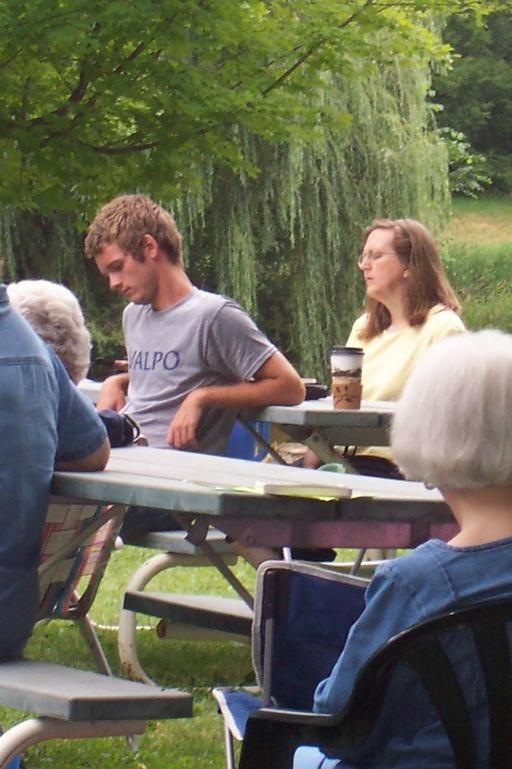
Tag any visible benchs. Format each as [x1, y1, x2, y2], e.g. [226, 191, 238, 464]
[0, 657, 193, 769]
[123, 589, 253, 647]
[118, 528, 239, 686]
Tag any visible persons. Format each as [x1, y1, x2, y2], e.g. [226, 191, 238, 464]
[84, 194, 306, 542]
[291, 217, 468, 561]
[0, 283, 111, 663]
[5, 278, 93, 614]
[294, 328, 511, 769]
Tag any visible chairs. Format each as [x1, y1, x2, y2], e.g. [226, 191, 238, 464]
[238, 594, 512, 769]
[205, 559, 371, 769]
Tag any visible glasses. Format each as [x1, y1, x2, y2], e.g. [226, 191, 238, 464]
[357, 249, 399, 268]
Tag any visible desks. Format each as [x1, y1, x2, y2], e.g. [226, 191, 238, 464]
[37, 443, 462, 756]
[238, 392, 399, 577]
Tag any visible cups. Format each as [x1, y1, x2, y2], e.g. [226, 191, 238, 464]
[329, 347, 365, 409]
